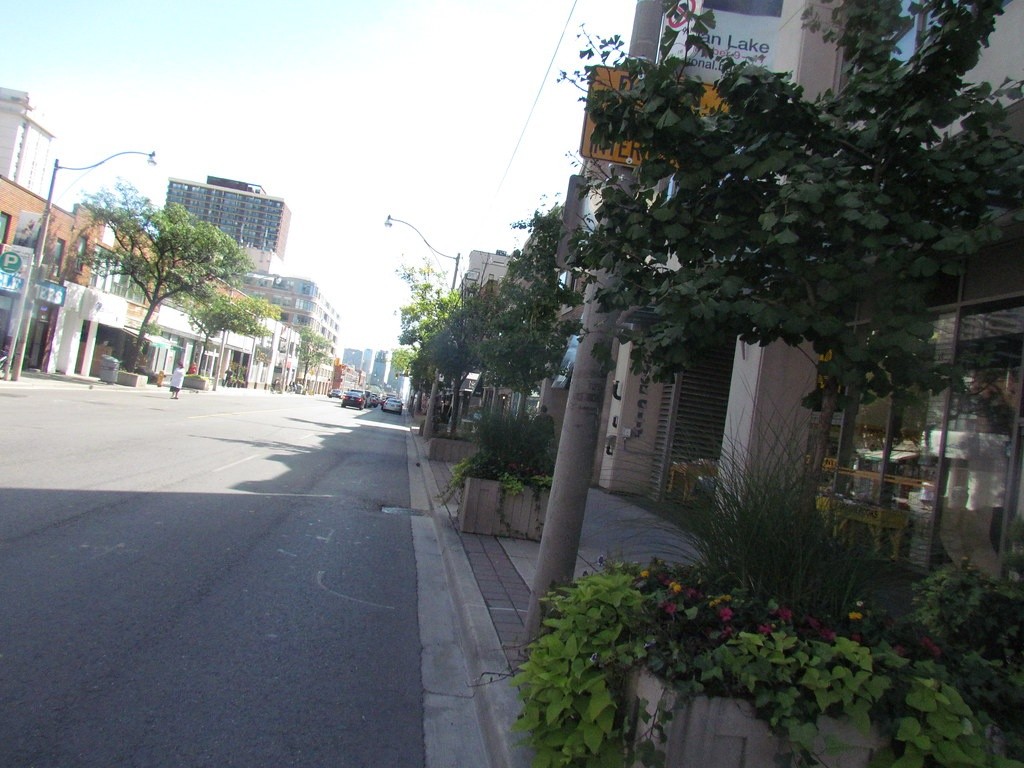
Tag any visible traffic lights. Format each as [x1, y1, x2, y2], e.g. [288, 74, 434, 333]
[395, 373, 399, 380]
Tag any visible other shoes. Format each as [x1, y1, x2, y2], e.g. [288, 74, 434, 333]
[175, 396, 178, 399]
[170, 397, 174, 399]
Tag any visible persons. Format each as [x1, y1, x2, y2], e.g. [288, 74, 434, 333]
[532, 404, 556, 453]
[272, 377, 295, 394]
[223, 367, 233, 388]
[169, 362, 185, 399]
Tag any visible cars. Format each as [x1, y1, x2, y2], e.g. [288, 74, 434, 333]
[340, 391, 366, 410]
[382, 397, 404, 416]
[350, 389, 399, 408]
[328, 388, 342, 398]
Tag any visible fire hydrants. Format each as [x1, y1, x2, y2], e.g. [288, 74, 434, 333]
[154, 370, 165, 388]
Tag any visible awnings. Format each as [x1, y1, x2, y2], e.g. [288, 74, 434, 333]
[123, 327, 185, 351]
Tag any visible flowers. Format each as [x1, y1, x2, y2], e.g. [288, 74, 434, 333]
[506, 359, 1018, 768]
[429, 397, 555, 539]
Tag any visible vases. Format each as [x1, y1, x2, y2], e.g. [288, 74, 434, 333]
[456, 475, 550, 544]
[622, 664, 885, 768]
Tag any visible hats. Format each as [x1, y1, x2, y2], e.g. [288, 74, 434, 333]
[176, 360, 183, 365]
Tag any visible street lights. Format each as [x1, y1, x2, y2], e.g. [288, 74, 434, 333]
[9, 152, 157, 382]
[385, 213, 463, 445]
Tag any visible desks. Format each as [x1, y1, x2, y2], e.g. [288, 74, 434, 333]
[665, 461, 719, 504]
